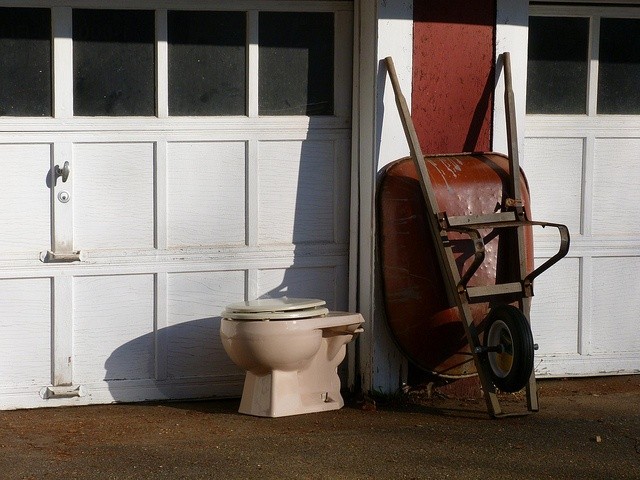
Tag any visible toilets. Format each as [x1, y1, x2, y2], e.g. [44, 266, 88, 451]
[220, 298, 364, 418]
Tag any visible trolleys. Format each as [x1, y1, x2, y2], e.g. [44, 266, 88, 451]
[377, 54, 570, 417]
